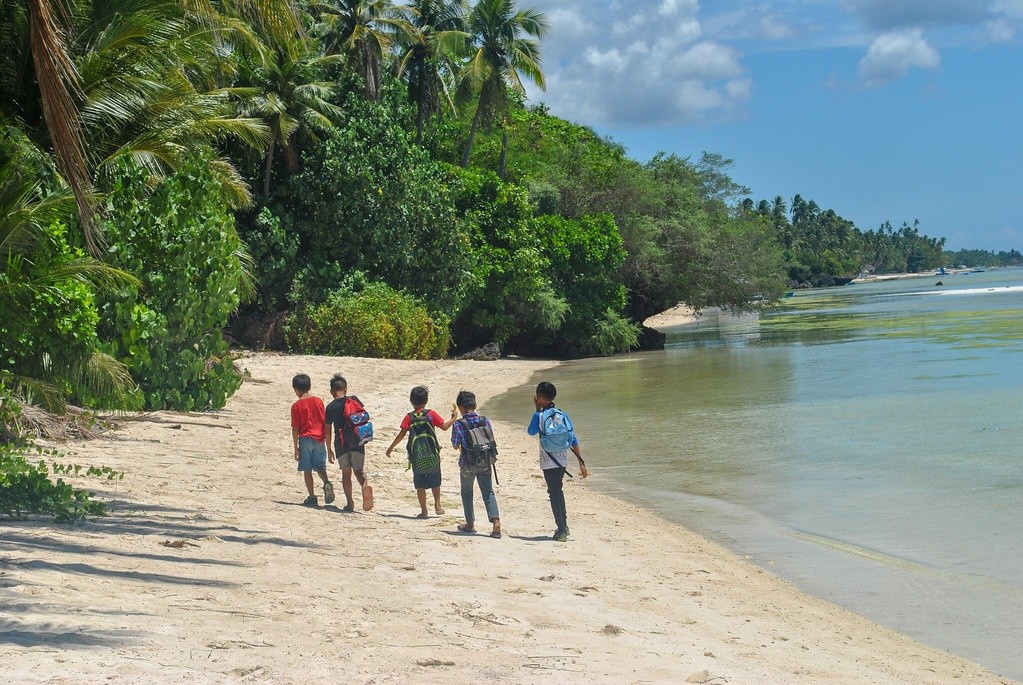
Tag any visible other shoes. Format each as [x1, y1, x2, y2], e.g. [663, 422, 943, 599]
[323, 481, 335, 504]
[362, 486, 373, 511]
[436, 508, 446, 515]
[555, 526, 570, 535]
[304, 496, 318, 506]
[344, 506, 354, 511]
[417, 514, 427, 518]
[553, 530, 567, 540]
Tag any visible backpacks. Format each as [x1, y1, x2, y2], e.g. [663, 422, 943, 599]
[405, 409, 442, 475]
[338, 398, 373, 448]
[456, 416, 499, 467]
[538, 402, 575, 452]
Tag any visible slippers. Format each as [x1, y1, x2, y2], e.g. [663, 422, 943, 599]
[490, 533, 501, 537]
[457, 524, 477, 532]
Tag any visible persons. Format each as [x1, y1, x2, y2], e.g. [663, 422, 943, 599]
[324, 373, 373, 513]
[291, 374, 335, 508]
[527, 382, 589, 541]
[451, 391, 503, 539]
[385, 386, 458, 520]
